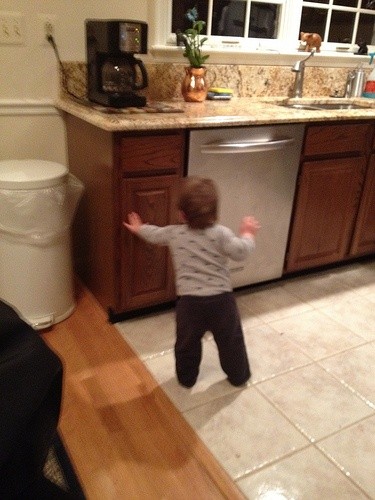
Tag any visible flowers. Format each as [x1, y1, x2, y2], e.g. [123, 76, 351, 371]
[174, 4, 211, 70]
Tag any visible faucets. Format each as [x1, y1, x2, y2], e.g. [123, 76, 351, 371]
[291, 48, 316, 98]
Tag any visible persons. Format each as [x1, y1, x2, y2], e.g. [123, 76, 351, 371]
[122, 176, 259, 388]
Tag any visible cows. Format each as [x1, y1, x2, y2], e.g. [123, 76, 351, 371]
[299, 29, 322, 54]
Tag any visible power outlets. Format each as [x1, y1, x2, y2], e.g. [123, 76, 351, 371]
[0, 10, 25, 47]
[39, 13, 56, 47]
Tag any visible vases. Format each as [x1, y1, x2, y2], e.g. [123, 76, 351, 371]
[182, 66, 216, 102]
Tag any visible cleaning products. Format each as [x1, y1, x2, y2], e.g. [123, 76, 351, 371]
[362, 51, 375, 99]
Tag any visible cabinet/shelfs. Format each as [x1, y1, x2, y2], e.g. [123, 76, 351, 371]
[63, 112, 375, 324]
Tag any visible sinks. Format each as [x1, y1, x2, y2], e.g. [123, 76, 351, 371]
[312, 100, 375, 109]
[262, 100, 320, 110]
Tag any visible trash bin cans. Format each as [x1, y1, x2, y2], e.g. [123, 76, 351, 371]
[0, 159, 78, 331]
